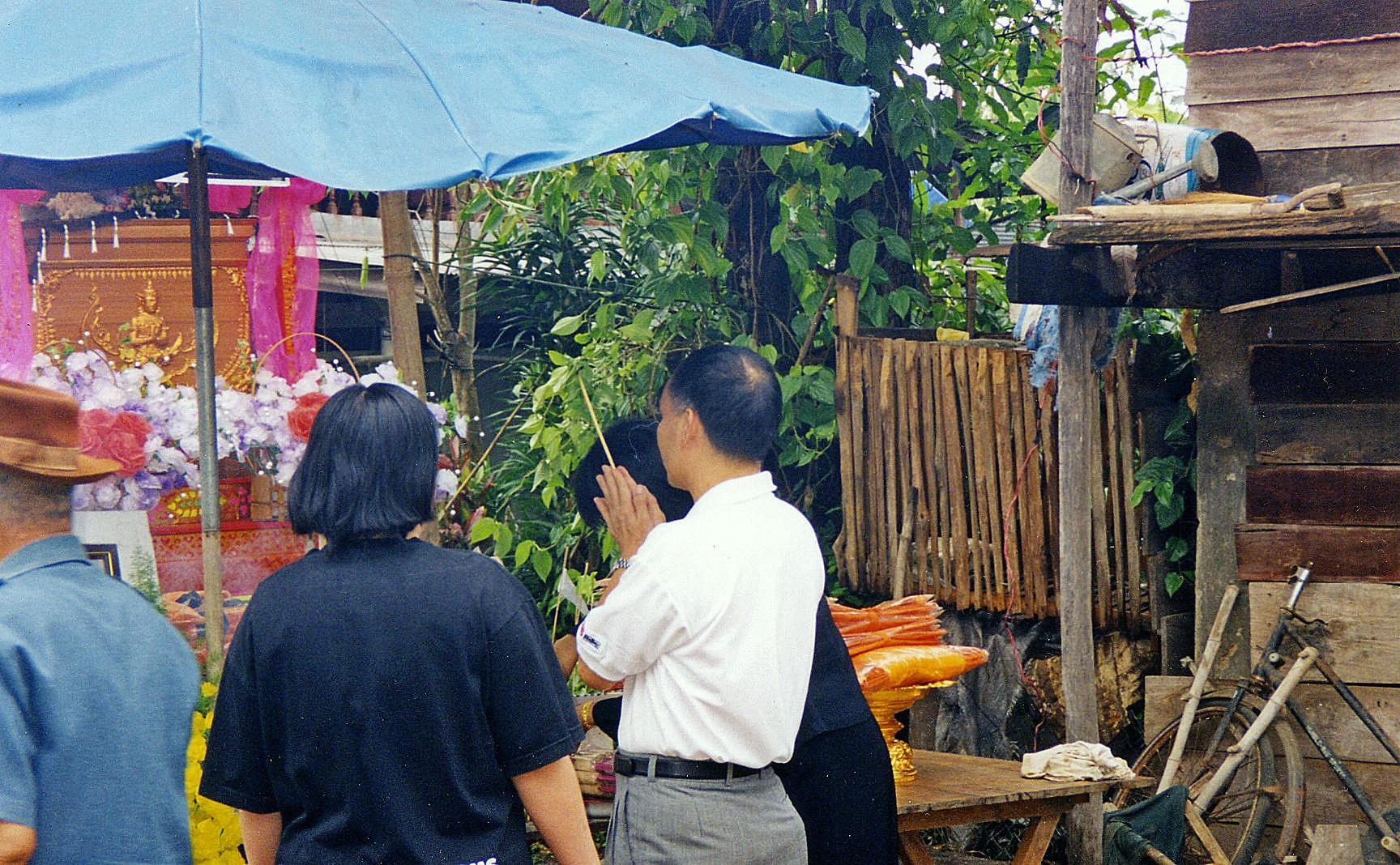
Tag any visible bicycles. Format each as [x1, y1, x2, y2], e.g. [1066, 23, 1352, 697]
[1110, 557, 1400, 865]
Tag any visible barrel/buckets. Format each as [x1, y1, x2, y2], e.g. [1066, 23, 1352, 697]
[1123, 119, 1263, 199]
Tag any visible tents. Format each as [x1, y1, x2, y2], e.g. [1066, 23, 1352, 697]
[0, 0, 880, 682]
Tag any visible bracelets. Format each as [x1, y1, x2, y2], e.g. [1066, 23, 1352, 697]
[582, 700, 597, 729]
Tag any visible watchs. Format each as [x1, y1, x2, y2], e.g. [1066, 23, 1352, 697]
[609, 556, 630, 570]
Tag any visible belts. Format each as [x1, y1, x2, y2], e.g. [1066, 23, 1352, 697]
[614, 751, 770, 780]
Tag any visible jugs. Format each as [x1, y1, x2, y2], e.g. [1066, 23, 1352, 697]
[1022, 112, 1217, 198]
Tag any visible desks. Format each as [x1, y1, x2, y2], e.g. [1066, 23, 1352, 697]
[524, 747, 1154, 865]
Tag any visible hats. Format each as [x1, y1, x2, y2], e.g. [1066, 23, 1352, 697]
[0, 379, 123, 484]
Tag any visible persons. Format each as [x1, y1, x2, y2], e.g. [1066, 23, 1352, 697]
[551, 418, 899, 865]
[0, 377, 202, 865]
[194, 383, 603, 865]
[578, 345, 826, 865]
